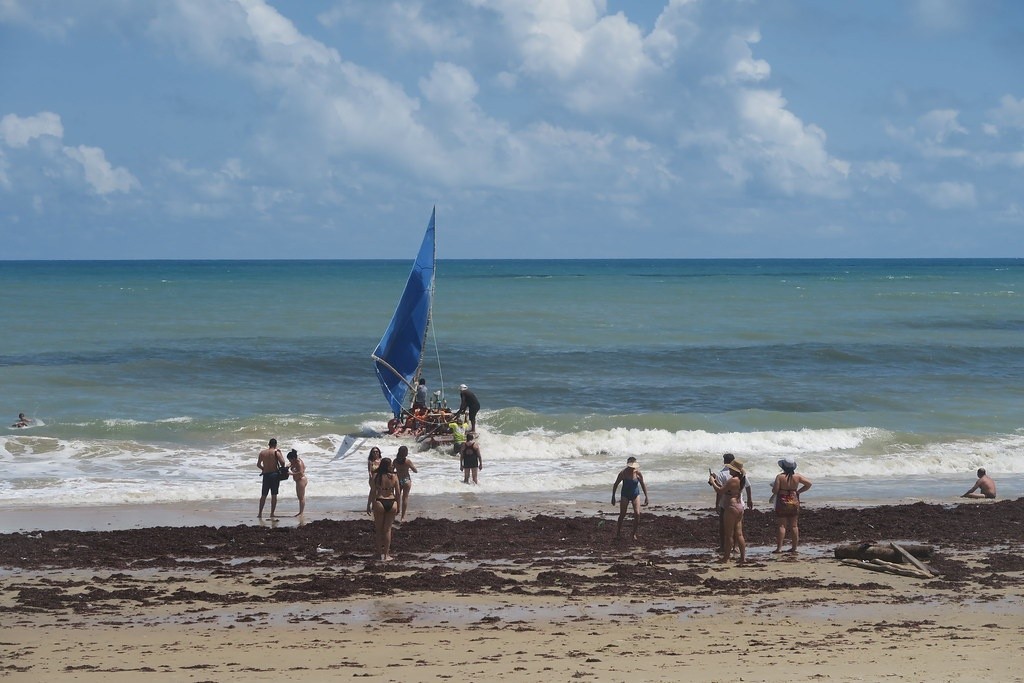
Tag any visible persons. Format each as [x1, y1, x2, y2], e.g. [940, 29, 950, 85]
[367, 447, 382, 512]
[414, 378, 428, 408]
[611, 457, 649, 540]
[257, 438, 285, 518]
[460, 433, 482, 484]
[456, 384, 480, 433]
[287, 449, 308, 518]
[709, 454, 753, 563]
[367, 458, 400, 562]
[772, 458, 812, 553]
[393, 446, 418, 521]
[18, 413, 32, 427]
[961, 468, 996, 499]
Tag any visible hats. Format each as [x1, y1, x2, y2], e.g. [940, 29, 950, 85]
[778, 457, 797, 471]
[724, 459, 745, 475]
[19, 413, 24, 417]
[458, 384, 468, 391]
[626, 460, 640, 469]
[457, 417, 464, 423]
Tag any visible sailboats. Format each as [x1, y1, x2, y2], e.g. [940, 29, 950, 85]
[373, 204, 479, 452]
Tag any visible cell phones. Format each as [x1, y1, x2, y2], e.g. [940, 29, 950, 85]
[709, 468, 711, 476]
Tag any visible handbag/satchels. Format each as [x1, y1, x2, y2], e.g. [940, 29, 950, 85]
[274, 448, 289, 480]
